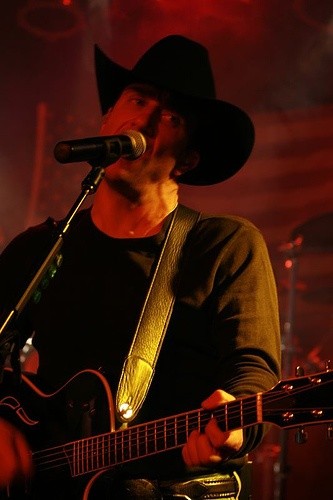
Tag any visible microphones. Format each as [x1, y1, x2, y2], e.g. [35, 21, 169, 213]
[52, 130, 146, 167]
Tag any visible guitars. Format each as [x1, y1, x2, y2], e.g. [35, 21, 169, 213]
[0, 366, 333, 500]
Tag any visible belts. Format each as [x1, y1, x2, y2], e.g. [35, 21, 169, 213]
[127, 474, 237, 500]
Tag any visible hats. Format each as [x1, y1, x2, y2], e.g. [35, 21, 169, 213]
[94, 35, 254, 186]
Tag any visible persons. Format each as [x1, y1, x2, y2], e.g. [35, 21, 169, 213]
[0, 31, 284, 499]
